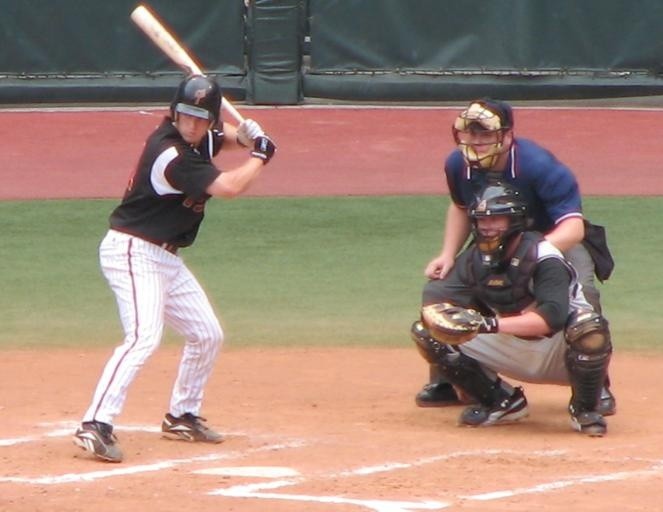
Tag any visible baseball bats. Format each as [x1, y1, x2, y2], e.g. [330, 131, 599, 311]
[129, 4, 243, 128]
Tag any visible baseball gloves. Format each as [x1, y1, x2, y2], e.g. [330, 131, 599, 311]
[420, 302, 483, 344]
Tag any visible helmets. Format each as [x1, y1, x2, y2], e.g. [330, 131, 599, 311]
[453, 95, 513, 172]
[170, 74, 220, 130]
[468, 181, 528, 267]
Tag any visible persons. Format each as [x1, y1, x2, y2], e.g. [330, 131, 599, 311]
[416, 97, 616, 416]
[71, 76, 277, 464]
[410, 181, 614, 436]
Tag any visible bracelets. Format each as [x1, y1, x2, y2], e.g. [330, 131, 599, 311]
[477, 313, 500, 335]
[249, 134, 276, 163]
[235, 130, 247, 153]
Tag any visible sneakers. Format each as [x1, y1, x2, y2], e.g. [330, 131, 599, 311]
[161, 412, 224, 444]
[571, 415, 607, 436]
[598, 398, 618, 416]
[414, 382, 459, 408]
[73, 421, 123, 462]
[458, 386, 529, 425]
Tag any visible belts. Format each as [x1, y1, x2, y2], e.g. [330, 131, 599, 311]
[109, 223, 177, 254]
